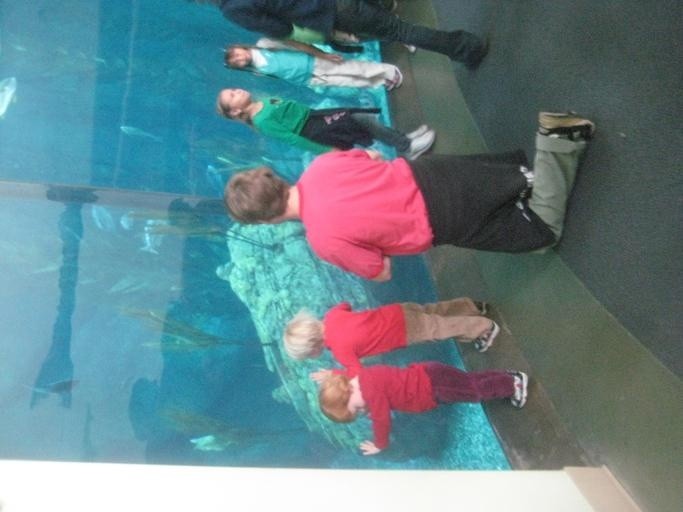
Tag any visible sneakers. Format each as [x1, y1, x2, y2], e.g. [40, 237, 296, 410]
[384, 80, 394, 91]
[405, 123, 427, 142]
[408, 130, 436, 162]
[473, 319, 499, 354]
[536, 107, 596, 144]
[464, 36, 489, 69]
[506, 368, 530, 408]
[472, 302, 489, 316]
[391, 64, 403, 88]
